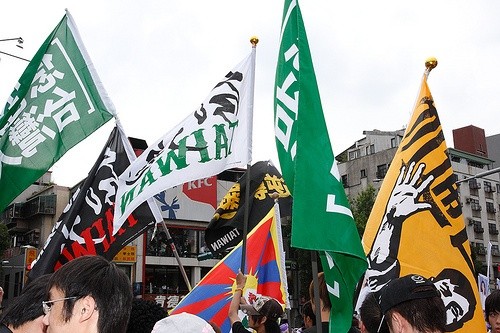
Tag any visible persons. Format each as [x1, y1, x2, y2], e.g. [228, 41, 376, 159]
[485, 289, 500, 333]
[42, 256, 132, 333]
[301, 272, 360, 333]
[0, 274, 52, 333]
[302, 301, 316, 329]
[125, 298, 168, 333]
[227, 268, 284, 333]
[377, 274, 447, 333]
[358, 291, 390, 333]
[480, 281, 487, 299]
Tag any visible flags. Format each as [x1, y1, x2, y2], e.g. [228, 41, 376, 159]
[112, 51, 255, 236]
[353, 73, 487, 333]
[274, 0, 369, 333]
[0, 11, 116, 216]
[27, 118, 163, 281]
[168, 203, 291, 333]
[205, 161, 293, 258]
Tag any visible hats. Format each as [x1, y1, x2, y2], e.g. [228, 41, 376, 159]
[238, 298, 284, 316]
[151, 312, 216, 333]
[377, 274, 442, 333]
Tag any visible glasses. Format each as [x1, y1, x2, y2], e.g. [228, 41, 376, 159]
[42, 297, 77, 314]
